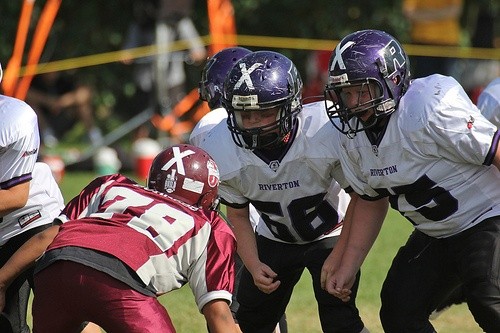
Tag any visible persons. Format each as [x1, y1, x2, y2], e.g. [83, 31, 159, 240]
[0, 62, 66, 333]
[0, 143, 244, 333]
[477, 76, 499, 126]
[400, 1, 499, 90]
[24, 34, 210, 149]
[325, 30, 500, 333]
[199, 51, 373, 333]
[186, 47, 253, 147]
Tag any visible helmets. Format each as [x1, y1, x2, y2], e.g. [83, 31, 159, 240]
[325, 29, 410, 135]
[221, 51, 304, 151]
[197, 47, 252, 105]
[151, 144, 222, 213]
